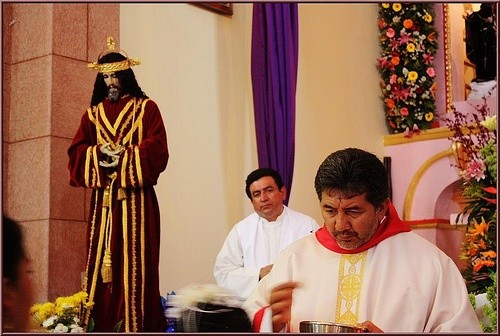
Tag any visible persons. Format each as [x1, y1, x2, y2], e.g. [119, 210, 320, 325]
[1, 215, 49, 334]
[166, 283, 251, 335]
[240, 148, 483, 334]
[66, 52, 169, 336]
[213, 168, 320, 305]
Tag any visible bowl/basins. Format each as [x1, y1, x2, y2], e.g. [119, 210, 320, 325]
[299, 321, 369, 333]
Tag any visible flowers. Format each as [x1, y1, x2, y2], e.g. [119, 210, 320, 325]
[376, 2, 438, 139]
[30, 291, 95, 334]
[438, 84, 497, 334]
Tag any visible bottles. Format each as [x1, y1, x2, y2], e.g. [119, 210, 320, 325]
[162, 292, 181, 333]
